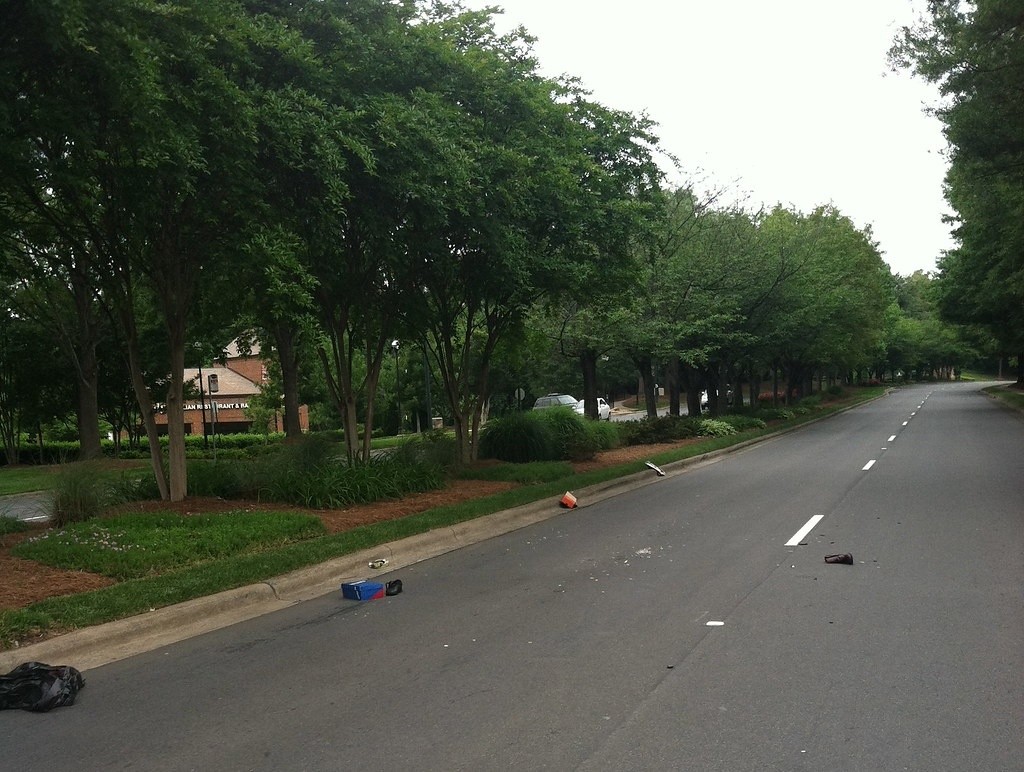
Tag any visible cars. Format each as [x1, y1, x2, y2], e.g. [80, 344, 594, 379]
[572, 397, 613, 422]
[757, 379, 798, 402]
[700, 384, 734, 411]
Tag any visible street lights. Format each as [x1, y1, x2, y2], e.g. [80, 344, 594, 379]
[193, 341, 210, 450]
[391, 340, 405, 437]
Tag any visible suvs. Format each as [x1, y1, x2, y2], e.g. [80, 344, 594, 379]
[532, 392, 579, 414]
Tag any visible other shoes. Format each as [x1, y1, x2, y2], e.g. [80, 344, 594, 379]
[386, 579, 402, 595]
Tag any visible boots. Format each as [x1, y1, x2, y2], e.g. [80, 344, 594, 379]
[825, 553, 854, 565]
